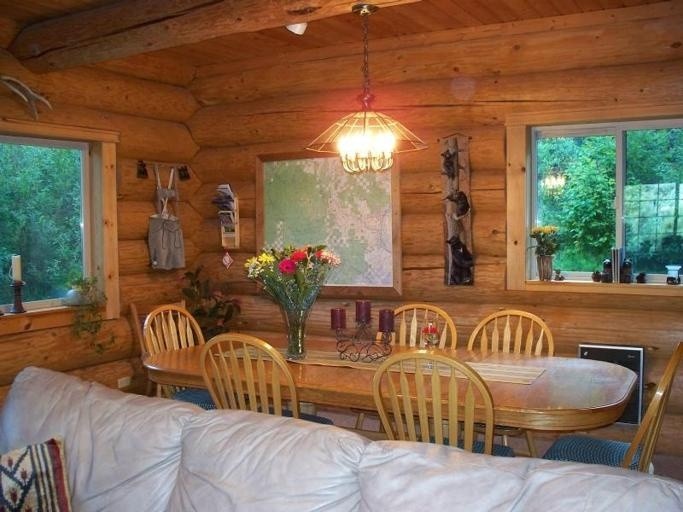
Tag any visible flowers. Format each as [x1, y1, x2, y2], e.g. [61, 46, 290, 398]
[529, 225, 559, 255]
[245, 242, 341, 356]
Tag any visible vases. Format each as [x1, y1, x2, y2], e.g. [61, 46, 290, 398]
[280, 294, 310, 363]
[536, 251, 555, 283]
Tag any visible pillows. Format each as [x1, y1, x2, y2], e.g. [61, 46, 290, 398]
[0, 432, 73, 512]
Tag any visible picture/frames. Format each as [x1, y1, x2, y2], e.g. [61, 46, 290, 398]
[254, 149, 404, 299]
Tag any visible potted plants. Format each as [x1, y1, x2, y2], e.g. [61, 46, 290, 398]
[65, 277, 116, 358]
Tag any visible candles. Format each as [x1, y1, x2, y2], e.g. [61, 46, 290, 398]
[10, 254, 22, 283]
[354, 297, 372, 323]
[377, 308, 395, 333]
[328, 305, 347, 332]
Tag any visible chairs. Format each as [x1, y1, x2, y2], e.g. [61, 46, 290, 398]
[467, 310, 554, 455]
[129, 299, 186, 396]
[199, 332, 333, 426]
[543, 338, 682, 476]
[349, 301, 462, 435]
[372, 347, 516, 456]
[138, 302, 260, 411]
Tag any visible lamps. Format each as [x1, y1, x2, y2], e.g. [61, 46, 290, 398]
[303, 4, 431, 174]
[541, 163, 568, 195]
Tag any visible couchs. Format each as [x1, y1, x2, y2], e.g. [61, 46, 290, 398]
[1, 366, 683, 512]
